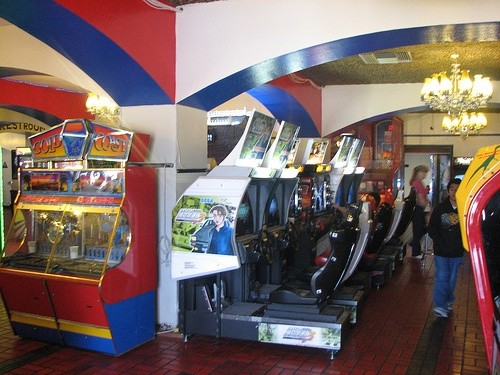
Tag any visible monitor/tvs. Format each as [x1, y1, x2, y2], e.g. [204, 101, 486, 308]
[288, 192, 295, 217]
[268, 196, 280, 227]
[235, 194, 254, 238]
[334, 185, 342, 207]
[347, 184, 354, 203]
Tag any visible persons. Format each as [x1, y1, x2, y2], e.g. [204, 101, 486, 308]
[409, 165, 430, 259]
[427, 179, 464, 318]
[333, 192, 381, 239]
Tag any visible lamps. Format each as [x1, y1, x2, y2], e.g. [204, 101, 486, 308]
[421, 54, 494, 141]
[86, 90, 120, 124]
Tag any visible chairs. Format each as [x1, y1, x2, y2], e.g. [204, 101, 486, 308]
[268, 187, 416, 314]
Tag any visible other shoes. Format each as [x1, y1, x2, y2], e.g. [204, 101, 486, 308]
[433, 305, 453, 317]
[412, 252, 425, 258]
